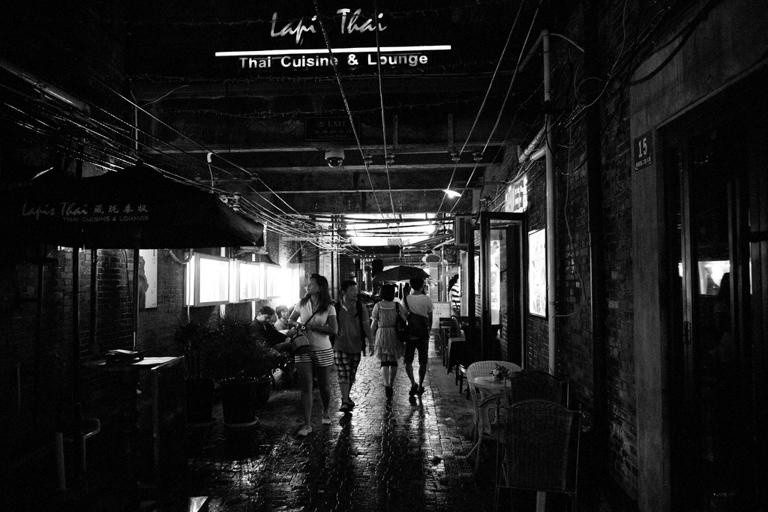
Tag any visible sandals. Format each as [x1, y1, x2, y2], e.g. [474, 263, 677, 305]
[339, 396, 354, 411]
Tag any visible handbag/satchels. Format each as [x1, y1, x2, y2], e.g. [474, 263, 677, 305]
[395, 316, 408, 341]
[408, 311, 429, 339]
[291, 331, 309, 355]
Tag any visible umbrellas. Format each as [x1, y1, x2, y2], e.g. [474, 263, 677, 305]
[375, 265, 430, 282]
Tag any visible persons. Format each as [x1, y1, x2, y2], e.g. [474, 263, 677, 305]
[251, 276, 434, 438]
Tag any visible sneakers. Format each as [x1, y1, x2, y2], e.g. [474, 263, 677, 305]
[409, 384, 425, 396]
[321, 414, 330, 424]
[385, 384, 393, 399]
[299, 425, 312, 435]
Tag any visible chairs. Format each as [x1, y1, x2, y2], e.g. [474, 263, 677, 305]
[436, 317, 582, 512]
[48, 319, 293, 512]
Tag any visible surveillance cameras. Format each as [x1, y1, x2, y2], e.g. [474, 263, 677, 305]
[325, 150, 345, 168]
